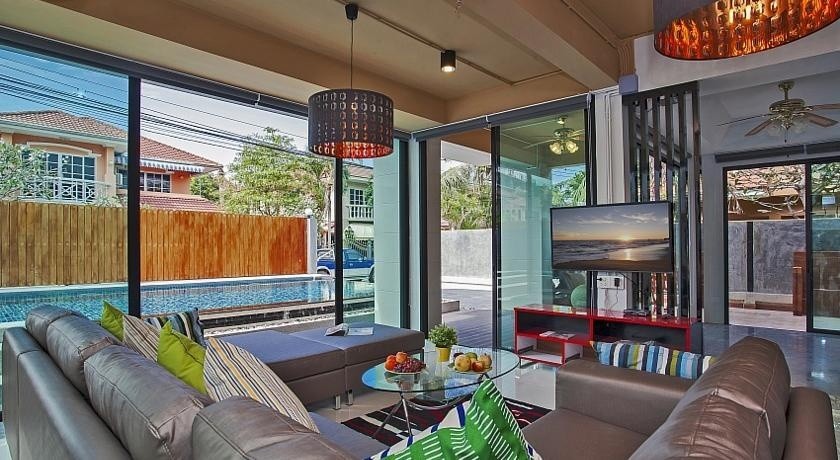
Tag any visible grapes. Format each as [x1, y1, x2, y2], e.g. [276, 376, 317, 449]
[393, 358, 426, 373]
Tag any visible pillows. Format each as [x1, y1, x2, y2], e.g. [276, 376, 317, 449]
[588, 340, 717, 379]
[101, 300, 125, 341]
[141, 308, 205, 347]
[123, 314, 160, 362]
[364, 378, 543, 460]
[203, 337, 320, 435]
[158, 319, 208, 395]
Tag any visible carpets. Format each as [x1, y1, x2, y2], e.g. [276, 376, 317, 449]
[342, 394, 553, 447]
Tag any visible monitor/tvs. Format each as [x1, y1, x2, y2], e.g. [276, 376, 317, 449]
[550, 199, 675, 272]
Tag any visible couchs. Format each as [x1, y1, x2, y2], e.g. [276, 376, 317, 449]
[2, 304, 838, 460]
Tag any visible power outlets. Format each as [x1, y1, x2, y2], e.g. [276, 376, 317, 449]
[597, 276, 626, 290]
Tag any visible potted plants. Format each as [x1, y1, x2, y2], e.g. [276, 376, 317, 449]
[429, 321, 459, 363]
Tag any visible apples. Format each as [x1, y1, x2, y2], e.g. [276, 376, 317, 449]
[455, 352, 491, 372]
[384, 352, 408, 370]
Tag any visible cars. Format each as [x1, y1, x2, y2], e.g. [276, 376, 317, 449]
[317, 248, 375, 284]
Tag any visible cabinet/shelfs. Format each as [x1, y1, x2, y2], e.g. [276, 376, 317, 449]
[514, 303, 697, 366]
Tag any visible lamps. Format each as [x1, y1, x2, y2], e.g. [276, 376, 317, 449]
[779, 113, 804, 158]
[651, 0, 840, 61]
[549, 134, 579, 155]
[308, 4, 395, 158]
[441, 50, 456, 73]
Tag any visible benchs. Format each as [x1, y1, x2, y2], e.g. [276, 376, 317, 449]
[218, 330, 346, 410]
[288, 322, 425, 404]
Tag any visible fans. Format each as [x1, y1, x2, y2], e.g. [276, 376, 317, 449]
[524, 115, 585, 149]
[715, 80, 840, 136]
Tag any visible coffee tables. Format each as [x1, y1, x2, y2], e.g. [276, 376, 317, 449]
[362, 347, 520, 438]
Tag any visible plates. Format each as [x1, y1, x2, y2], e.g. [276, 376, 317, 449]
[447, 363, 492, 374]
[384, 367, 425, 374]
